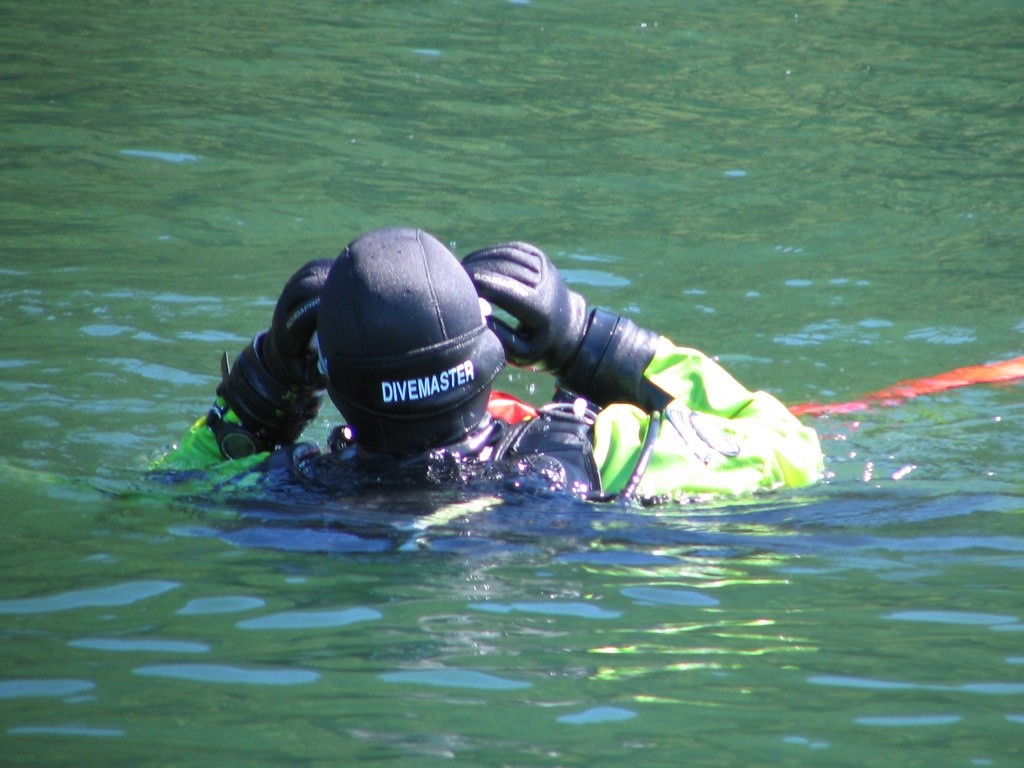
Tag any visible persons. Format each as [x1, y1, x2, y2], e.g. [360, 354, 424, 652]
[160, 228, 822, 502]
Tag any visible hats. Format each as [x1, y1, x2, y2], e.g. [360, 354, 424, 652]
[315, 228, 506, 456]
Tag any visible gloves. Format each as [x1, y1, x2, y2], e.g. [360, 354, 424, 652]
[216, 256, 336, 451]
[460, 242, 672, 417]
